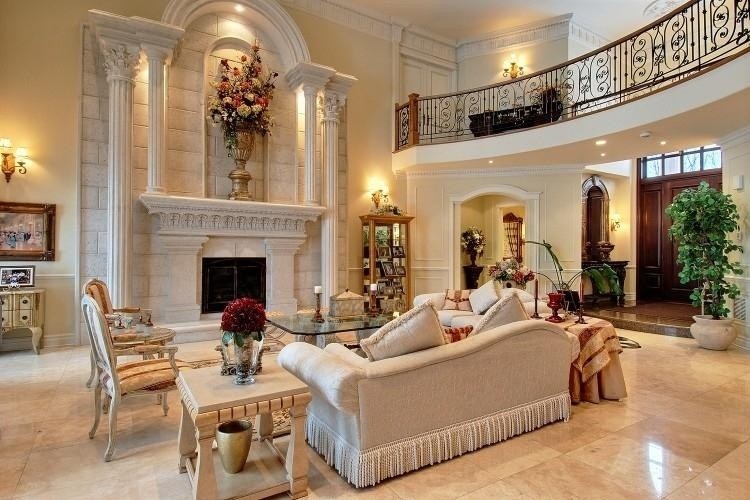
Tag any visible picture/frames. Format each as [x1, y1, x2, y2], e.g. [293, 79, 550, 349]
[1, 265, 36, 287]
[0, 202, 57, 264]
[376, 245, 405, 300]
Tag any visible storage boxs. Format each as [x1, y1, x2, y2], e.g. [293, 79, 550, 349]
[330, 287, 366, 318]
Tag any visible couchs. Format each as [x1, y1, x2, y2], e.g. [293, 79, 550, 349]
[412, 285, 548, 330]
[279, 322, 580, 487]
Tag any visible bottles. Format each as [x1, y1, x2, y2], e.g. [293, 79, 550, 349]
[134, 315, 155, 337]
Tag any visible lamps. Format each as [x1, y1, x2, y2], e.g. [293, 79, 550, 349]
[368, 182, 387, 209]
[499, 62, 526, 77]
[611, 214, 622, 232]
[0, 140, 30, 183]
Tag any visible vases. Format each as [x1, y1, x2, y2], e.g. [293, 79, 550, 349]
[471, 254, 478, 267]
[505, 282, 519, 289]
[604, 250, 611, 262]
[233, 339, 256, 383]
[224, 133, 256, 203]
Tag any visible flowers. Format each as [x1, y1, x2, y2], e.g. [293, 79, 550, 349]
[205, 35, 276, 135]
[489, 263, 534, 282]
[461, 227, 486, 256]
[219, 296, 271, 346]
[600, 242, 616, 248]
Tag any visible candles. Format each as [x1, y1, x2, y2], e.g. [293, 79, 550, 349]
[369, 283, 378, 291]
[313, 285, 322, 294]
[534, 277, 539, 299]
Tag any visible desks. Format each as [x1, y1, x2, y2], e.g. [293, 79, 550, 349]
[583, 260, 630, 309]
[101, 324, 178, 414]
[462, 264, 483, 289]
[467, 99, 564, 135]
[540, 313, 630, 404]
[174, 362, 313, 500]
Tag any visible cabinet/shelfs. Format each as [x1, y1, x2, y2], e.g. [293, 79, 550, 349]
[1, 287, 46, 354]
[499, 210, 524, 262]
[360, 215, 417, 313]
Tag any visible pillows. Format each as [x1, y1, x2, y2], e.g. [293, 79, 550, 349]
[469, 279, 499, 315]
[470, 293, 534, 336]
[440, 325, 473, 344]
[358, 302, 449, 362]
[440, 288, 472, 311]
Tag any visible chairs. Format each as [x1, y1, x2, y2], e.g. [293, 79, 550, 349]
[81, 296, 182, 462]
[80, 278, 171, 383]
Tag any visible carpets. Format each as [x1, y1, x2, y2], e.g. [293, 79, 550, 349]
[178, 351, 297, 453]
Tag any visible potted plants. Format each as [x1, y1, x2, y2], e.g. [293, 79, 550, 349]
[664, 178, 746, 352]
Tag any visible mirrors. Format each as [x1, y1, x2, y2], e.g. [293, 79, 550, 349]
[582, 175, 611, 246]
